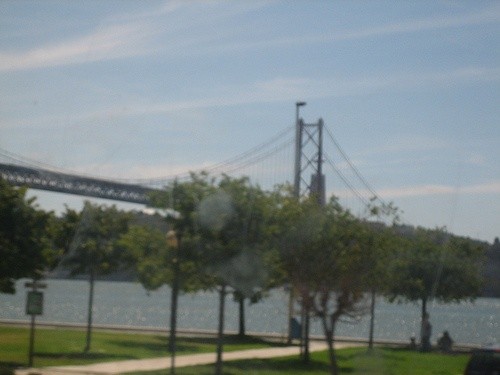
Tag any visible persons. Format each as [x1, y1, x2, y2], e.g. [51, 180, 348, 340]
[437, 331, 454, 349]
[422, 312, 433, 351]
[289, 318, 302, 340]
